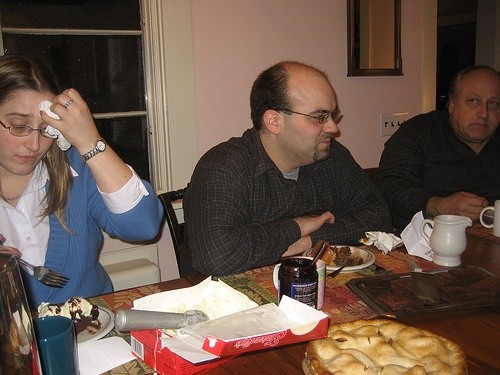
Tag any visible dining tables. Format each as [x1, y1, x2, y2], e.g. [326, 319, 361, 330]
[35, 206, 500, 375]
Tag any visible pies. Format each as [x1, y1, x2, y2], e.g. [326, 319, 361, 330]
[306, 316, 469, 375]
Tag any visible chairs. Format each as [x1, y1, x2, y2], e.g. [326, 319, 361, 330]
[158, 183, 194, 278]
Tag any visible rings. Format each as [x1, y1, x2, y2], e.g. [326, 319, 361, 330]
[65, 99, 73, 108]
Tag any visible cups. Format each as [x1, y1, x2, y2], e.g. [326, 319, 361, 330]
[272, 256, 326, 309]
[479, 200, 500, 238]
[32, 315, 79, 375]
[419, 214, 473, 267]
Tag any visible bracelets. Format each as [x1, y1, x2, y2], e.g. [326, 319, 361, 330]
[431, 195, 440, 217]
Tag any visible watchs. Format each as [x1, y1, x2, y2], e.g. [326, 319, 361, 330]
[80, 138, 107, 163]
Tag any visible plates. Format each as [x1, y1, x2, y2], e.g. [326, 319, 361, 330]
[484, 211, 494, 217]
[344, 264, 500, 315]
[302, 245, 376, 271]
[76, 306, 115, 346]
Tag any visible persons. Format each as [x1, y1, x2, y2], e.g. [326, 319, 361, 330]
[180, 61, 392, 277]
[0, 56, 165, 310]
[376, 65, 500, 232]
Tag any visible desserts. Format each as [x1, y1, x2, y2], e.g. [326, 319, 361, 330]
[310, 241, 362, 267]
[38, 297, 99, 334]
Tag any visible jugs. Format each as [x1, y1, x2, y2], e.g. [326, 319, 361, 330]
[0, 252, 43, 375]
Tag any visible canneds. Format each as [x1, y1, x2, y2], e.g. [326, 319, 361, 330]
[277, 257, 318, 311]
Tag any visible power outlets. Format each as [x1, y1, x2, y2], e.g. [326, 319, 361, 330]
[380, 111, 409, 136]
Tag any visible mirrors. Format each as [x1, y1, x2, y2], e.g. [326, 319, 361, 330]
[346, 0, 405, 77]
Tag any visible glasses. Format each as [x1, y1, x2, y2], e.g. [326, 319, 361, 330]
[271, 108, 342, 124]
[0, 120, 58, 139]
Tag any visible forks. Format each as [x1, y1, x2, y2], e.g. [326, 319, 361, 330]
[0, 240, 68, 287]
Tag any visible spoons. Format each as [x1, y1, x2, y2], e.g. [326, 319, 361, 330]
[327, 257, 363, 279]
